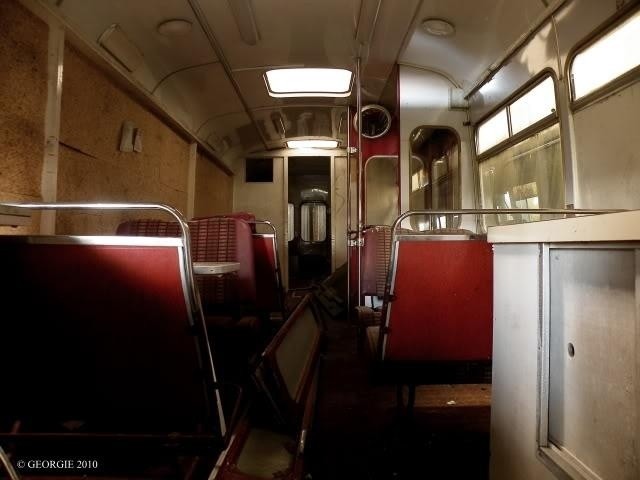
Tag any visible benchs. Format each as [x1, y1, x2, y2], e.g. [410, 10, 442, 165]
[367, 206, 632, 364]
[116, 218, 259, 338]
[1, 202, 246, 441]
[362, 227, 477, 296]
[191, 213, 288, 306]
